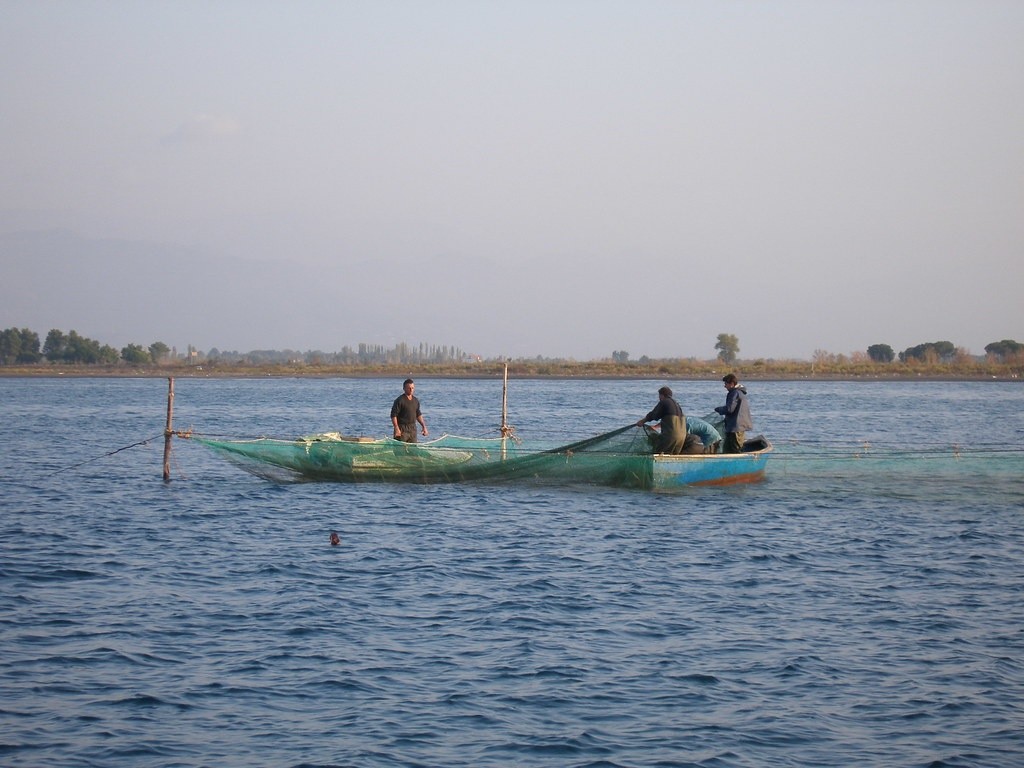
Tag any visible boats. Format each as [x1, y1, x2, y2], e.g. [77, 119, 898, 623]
[203, 432, 775, 494]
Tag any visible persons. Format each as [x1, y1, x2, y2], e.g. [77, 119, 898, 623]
[390, 380, 428, 456]
[685, 374, 753, 453]
[638, 386, 686, 455]
